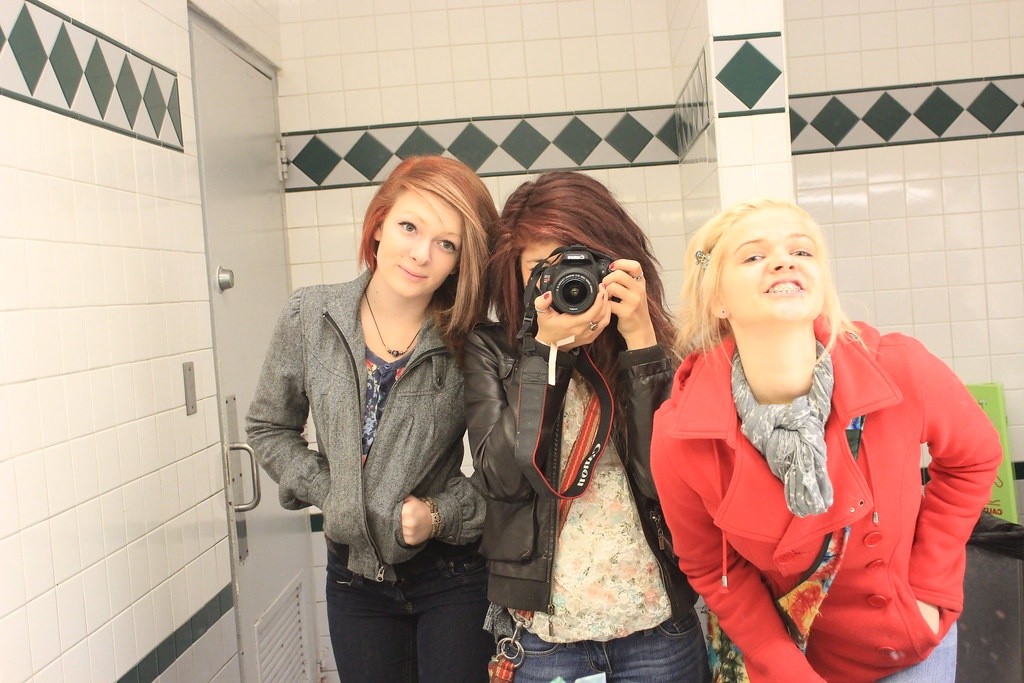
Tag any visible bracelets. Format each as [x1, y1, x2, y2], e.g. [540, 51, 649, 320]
[424, 496, 440, 541]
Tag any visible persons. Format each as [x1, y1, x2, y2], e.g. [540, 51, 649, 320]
[462, 171, 710, 683]
[244, 157, 497, 683]
[649, 200, 1003, 683]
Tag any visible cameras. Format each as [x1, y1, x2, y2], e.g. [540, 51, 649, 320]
[540, 243, 612, 314]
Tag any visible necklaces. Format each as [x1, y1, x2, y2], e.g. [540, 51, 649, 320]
[364, 290, 421, 356]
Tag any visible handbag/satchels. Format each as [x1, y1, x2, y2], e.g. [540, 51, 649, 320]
[697, 594, 824, 683]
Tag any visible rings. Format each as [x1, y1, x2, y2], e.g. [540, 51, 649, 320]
[590, 321, 599, 331]
[631, 271, 644, 280]
